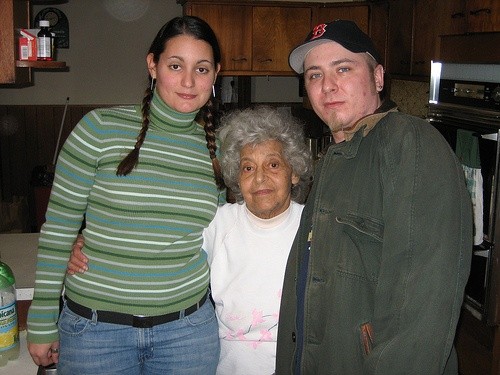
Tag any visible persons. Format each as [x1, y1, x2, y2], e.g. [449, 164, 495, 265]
[67, 104, 313, 375]
[275, 19, 475, 375]
[26, 17, 220, 374]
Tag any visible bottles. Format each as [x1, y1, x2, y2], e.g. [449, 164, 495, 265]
[0, 261, 19, 367]
[36, 20, 53, 61]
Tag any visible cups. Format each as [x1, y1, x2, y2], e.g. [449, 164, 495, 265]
[306, 133, 332, 159]
[50, 33, 57, 61]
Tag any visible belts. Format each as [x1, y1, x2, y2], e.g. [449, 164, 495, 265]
[65, 289, 209, 327]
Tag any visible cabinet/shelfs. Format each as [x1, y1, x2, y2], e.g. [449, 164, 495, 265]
[373, 0, 500, 82]
[176, 0, 371, 159]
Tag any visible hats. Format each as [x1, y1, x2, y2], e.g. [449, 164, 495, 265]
[288, 20, 378, 74]
